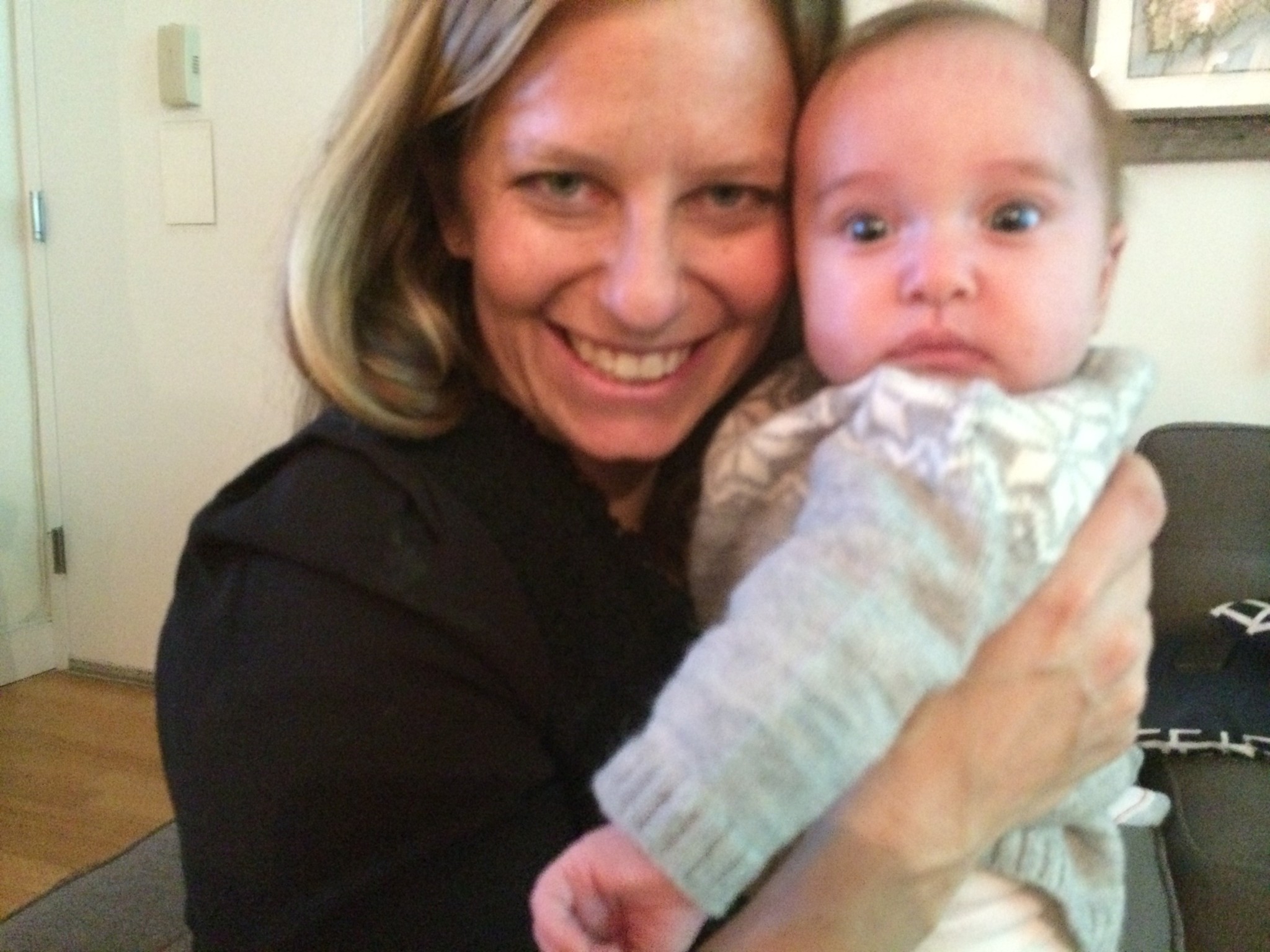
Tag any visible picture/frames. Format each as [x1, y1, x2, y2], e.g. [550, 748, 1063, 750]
[1044, 1, 1270, 162]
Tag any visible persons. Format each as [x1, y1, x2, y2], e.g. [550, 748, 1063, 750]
[154, 1, 1172, 952]
[527, 0, 1167, 952]
